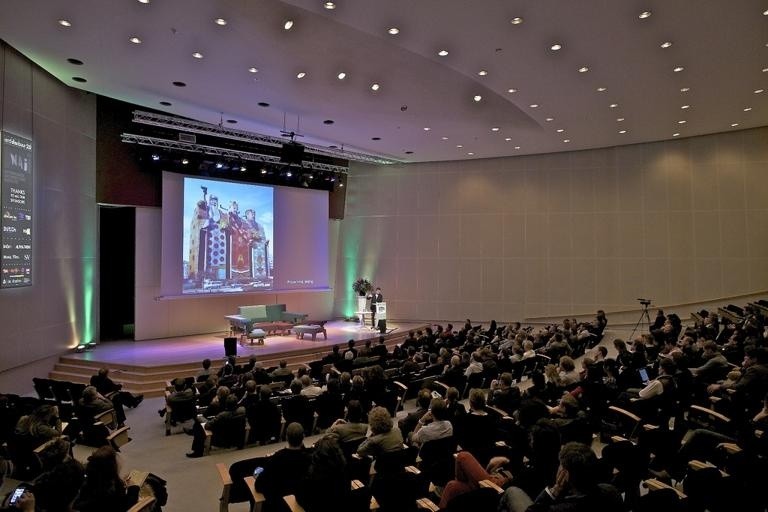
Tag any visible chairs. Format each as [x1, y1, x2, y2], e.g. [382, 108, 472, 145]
[0, 377, 157, 512]
[164, 345, 393, 512]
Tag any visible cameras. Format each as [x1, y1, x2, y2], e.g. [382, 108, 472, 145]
[636, 295, 653, 308]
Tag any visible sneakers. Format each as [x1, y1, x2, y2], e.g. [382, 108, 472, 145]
[132, 394, 144, 408]
[158, 410, 164, 416]
[117, 425, 131, 429]
[185, 452, 203, 457]
[183, 427, 194, 435]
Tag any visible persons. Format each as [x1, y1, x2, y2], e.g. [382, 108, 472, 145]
[159, 298, 767, 511]
[366, 287, 383, 330]
[243, 208, 270, 282]
[2, 368, 168, 511]
[189, 193, 230, 287]
[225, 200, 252, 283]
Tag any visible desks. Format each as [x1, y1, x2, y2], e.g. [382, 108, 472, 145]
[354, 312, 373, 329]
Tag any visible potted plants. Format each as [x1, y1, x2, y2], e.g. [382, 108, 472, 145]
[352, 278, 375, 312]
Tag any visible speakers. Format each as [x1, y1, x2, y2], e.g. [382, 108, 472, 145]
[223, 337, 238, 356]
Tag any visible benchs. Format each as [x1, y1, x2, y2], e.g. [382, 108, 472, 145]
[292, 320, 328, 341]
[240, 320, 266, 345]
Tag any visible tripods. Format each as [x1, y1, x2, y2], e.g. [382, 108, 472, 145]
[628, 309, 652, 340]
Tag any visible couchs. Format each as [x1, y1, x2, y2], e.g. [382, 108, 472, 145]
[224, 304, 308, 338]
